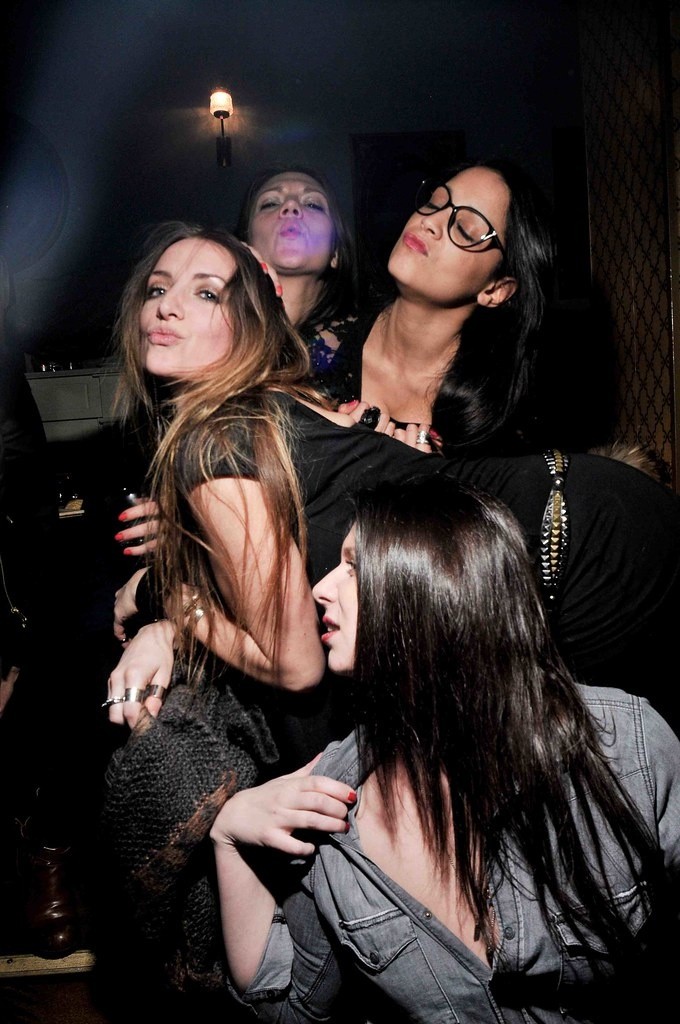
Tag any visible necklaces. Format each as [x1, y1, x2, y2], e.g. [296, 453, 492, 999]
[445, 848, 495, 970]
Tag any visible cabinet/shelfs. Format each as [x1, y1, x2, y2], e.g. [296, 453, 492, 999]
[25, 367, 134, 441]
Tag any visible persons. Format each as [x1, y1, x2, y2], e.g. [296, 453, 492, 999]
[0, 156, 680, 976]
[210, 466, 680, 1024]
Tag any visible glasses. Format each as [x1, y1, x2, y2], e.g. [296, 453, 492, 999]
[414, 175, 515, 272]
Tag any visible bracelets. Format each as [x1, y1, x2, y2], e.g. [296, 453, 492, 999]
[181, 593, 217, 639]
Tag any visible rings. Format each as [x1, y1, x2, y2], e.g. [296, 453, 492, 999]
[124, 688, 146, 704]
[103, 696, 126, 708]
[143, 684, 167, 704]
[119, 636, 129, 643]
[415, 431, 430, 444]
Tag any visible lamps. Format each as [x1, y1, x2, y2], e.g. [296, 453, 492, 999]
[209, 91, 234, 167]
